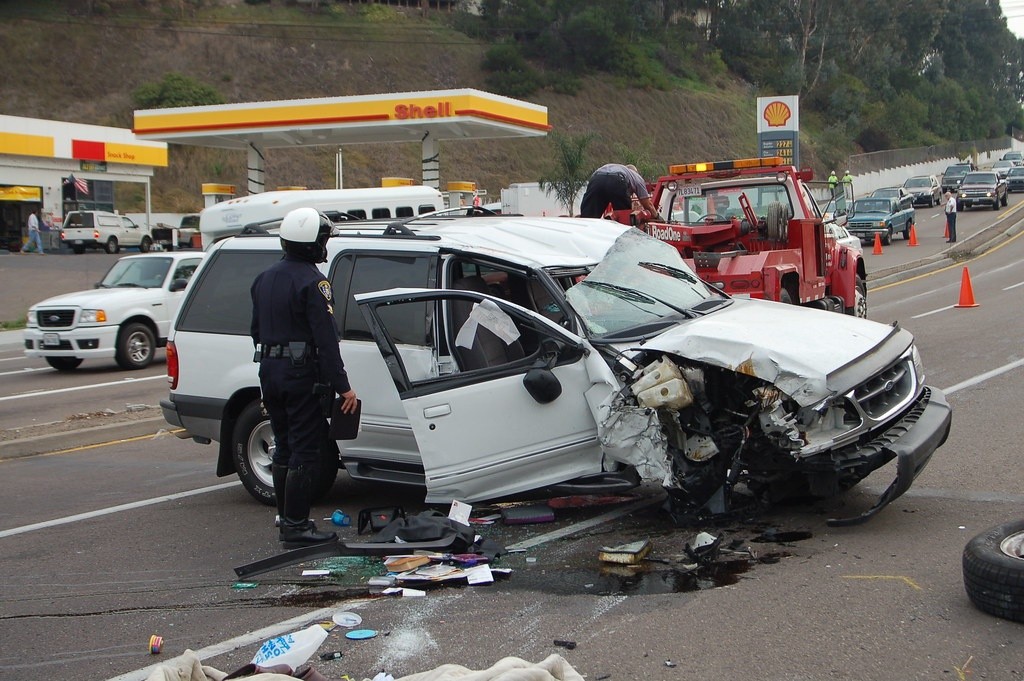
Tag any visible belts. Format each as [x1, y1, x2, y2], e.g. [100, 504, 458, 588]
[268, 346, 290, 357]
[596, 172, 623, 177]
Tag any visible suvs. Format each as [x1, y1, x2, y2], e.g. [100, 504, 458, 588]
[158, 184, 954, 525]
[20, 251, 209, 370]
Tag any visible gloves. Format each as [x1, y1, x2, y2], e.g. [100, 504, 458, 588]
[655, 213, 665, 223]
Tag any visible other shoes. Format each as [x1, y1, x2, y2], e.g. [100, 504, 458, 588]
[946, 240, 953, 243]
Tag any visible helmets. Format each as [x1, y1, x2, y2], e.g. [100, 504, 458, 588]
[846, 170, 850, 173]
[831, 171, 836, 175]
[280, 208, 340, 263]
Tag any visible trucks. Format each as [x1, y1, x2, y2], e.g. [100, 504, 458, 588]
[601, 157, 874, 330]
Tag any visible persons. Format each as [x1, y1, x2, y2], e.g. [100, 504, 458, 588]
[945, 193, 957, 242]
[581, 164, 664, 223]
[843, 170, 852, 199]
[20, 209, 45, 256]
[828, 171, 838, 198]
[250, 208, 358, 550]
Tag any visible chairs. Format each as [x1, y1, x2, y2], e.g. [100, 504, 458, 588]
[452, 274, 526, 372]
[508, 273, 571, 357]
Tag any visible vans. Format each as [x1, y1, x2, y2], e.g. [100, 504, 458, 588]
[60, 210, 155, 255]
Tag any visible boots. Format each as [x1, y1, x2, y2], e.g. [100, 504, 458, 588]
[283, 464, 338, 548]
[272, 463, 314, 541]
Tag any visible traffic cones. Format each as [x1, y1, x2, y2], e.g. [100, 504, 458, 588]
[906, 223, 919, 247]
[953, 266, 981, 309]
[871, 231, 885, 255]
[943, 220, 949, 240]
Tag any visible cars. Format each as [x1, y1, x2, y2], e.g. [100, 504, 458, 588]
[821, 196, 855, 223]
[866, 186, 917, 211]
[992, 159, 1018, 179]
[900, 174, 943, 207]
[956, 171, 1009, 211]
[844, 197, 916, 244]
[1005, 167, 1024, 194]
[940, 163, 974, 193]
[999, 151, 1024, 166]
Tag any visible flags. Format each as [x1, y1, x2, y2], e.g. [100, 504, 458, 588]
[64, 174, 88, 195]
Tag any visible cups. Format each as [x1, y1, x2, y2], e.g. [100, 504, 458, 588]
[331, 510, 351, 527]
